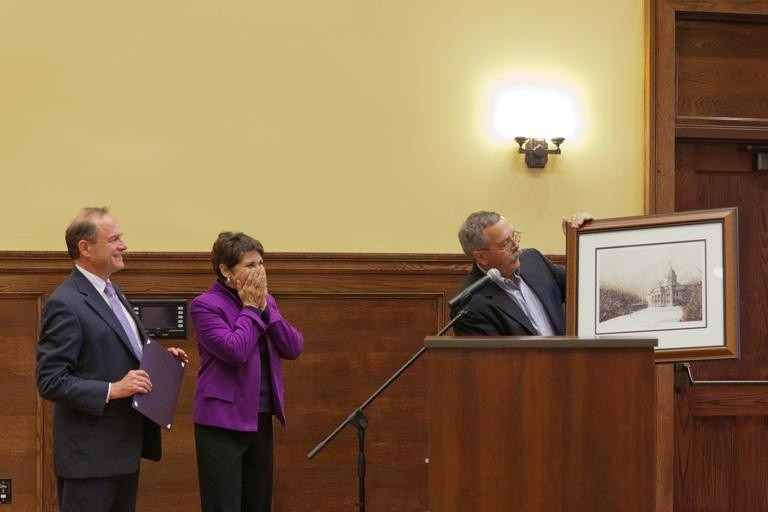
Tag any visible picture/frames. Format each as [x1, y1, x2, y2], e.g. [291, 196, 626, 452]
[563, 203, 744, 367]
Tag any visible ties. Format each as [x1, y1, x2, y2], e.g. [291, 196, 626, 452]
[104, 282, 143, 362]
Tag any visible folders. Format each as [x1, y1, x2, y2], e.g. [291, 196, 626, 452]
[132, 335, 186, 431]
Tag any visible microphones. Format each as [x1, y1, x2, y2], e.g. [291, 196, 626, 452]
[447, 268, 500, 308]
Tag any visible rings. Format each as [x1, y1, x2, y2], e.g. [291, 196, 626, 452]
[254, 283, 260, 289]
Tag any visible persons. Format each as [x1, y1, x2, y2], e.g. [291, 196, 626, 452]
[32, 208, 187, 510]
[188, 231, 304, 511]
[449, 210, 595, 340]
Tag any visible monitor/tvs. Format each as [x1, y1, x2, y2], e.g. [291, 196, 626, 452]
[130, 300, 187, 338]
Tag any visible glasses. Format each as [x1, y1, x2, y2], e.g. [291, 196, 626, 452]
[471, 230, 522, 257]
